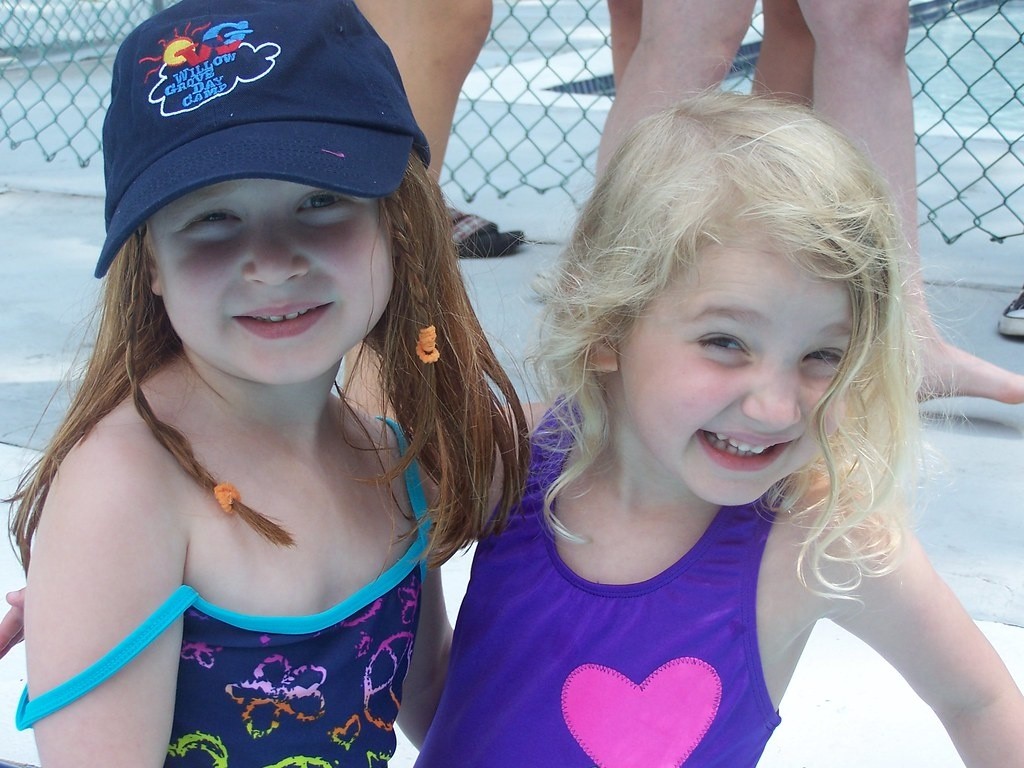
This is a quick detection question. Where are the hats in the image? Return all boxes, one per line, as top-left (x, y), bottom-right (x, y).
top-left (91, 1), bottom-right (429, 279)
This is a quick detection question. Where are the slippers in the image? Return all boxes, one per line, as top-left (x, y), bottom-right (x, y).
top-left (447, 213), bottom-right (527, 260)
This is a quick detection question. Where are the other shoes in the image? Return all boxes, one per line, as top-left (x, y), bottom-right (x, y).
top-left (996, 293), bottom-right (1024, 338)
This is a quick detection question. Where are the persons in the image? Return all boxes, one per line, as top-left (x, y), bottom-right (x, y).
top-left (0, 89), bottom-right (1024, 768)
top-left (593, 0), bottom-right (757, 190)
top-left (751, 1), bottom-right (1023, 404)
top-left (350, 0), bottom-right (528, 261)
top-left (9, 1), bottom-right (540, 768)
